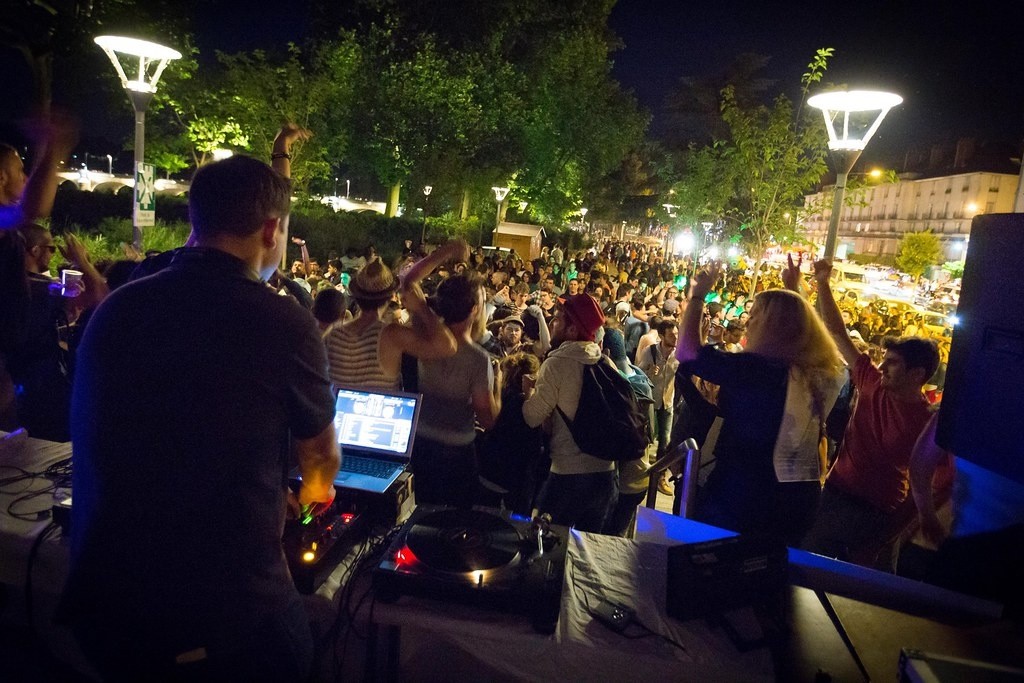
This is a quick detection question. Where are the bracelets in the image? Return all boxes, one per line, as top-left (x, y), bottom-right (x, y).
top-left (692, 296), bottom-right (705, 302)
top-left (271, 151), bottom-right (292, 160)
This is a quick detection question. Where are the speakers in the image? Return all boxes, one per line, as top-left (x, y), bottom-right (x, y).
top-left (934, 212), bottom-right (1024, 487)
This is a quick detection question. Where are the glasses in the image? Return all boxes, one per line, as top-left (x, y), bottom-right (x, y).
top-left (25, 244), bottom-right (57, 254)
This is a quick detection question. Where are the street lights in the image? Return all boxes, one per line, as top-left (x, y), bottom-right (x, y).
top-left (87, 35), bottom-right (183, 257)
top-left (577, 206), bottom-right (589, 227)
top-left (104, 153), bottom-right (113, 177)
top-left (345, 180), bottom-right (351, 200)
top-left (420, 185), bottom-right (434, 248)
top-left (491, 187), bottom-right (514, 249)
top-left (804, 88), bottom-right (904, 312)
top-left (700, 218), bottom-right (712, 261)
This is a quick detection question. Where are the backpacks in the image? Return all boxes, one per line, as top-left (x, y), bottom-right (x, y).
top-left (555, 354), bottom-right (652, 461)
top-left (603, 301), bottom-right (629, 324)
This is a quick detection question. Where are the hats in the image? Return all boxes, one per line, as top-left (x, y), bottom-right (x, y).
top-left (347, 256), bottom-right (401, 301)
top-left (554, 291), bottom-right (606, 342)
top-left (663, 299), bottom-right (680, 311)
top-left (502, 315), bottom-right (526, 329)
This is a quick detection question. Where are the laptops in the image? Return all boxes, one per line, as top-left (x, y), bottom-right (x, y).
top-left (287, 382), bottom-right (424, 493)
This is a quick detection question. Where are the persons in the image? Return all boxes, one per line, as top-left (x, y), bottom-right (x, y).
top-left (266, 121), bottom-right (1024, 606)
top-left (0, 106), bottom-right (135, 441)
top-left (72, 155), bottom-right (341, 683)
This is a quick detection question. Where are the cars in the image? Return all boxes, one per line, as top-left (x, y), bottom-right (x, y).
top-left (828, 262), bottom-right (962, 349)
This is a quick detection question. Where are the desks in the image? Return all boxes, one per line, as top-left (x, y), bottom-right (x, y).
top-left (0, 429), bottom-right (1024, 683)
